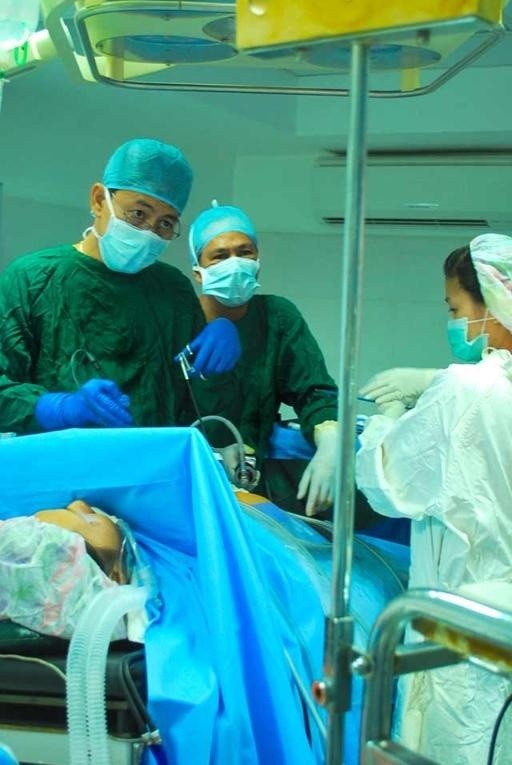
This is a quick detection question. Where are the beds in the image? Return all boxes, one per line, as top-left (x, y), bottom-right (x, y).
top-left (2, 621), bottom-right (146, 765)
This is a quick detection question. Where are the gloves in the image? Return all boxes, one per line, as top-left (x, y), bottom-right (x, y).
top-left (296, 420), bottom-right (338, 517)
top-left (359, 367), bottom-right (437, 407)
top-left (35, 378), bottom-right (135, 431)
top-left (173, 316), bottom-right (242, 379)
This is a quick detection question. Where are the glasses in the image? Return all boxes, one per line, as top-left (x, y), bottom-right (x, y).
top-left (112, 192), bottom-right (181, 241)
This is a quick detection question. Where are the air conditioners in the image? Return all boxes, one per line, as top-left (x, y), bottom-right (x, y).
top-left (314, 154), bottom-right (510, 238)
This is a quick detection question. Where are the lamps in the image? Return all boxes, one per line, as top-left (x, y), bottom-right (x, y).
top-left (40, 0), bottom-right (504, 98)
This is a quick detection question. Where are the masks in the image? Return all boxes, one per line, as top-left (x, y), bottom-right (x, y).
top-left (199, 260), bottom-right (262, 308)
top-left (447, 320), bottom-right (489, 364)
top-left (95, 218), bottom-right (172, 274)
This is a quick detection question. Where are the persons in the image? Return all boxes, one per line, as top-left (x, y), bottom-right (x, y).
top-left (1, 139), bottom-right (246, 434)
top-left (355, 231), bottom-right (512, 765)
top-left (0, 498), bottom-right (144, 647)
top-left (187, 207), bottom-right (338, 518)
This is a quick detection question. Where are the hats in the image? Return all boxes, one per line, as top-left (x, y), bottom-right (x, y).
top-left (103, 138), bottom-right (194, 211)
top-left (188, 205), bottom-right (259, 268)
